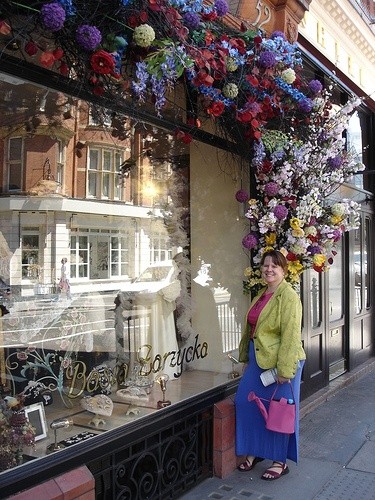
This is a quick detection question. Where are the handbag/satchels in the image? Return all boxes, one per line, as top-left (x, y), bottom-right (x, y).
top-left (249, 381), bottom-right (295, 434)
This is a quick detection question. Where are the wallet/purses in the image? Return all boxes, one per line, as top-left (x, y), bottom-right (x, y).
top-left (260, 368), bottom-right (277, 387)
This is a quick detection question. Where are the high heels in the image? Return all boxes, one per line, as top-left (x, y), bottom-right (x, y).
top-left (238, 456), bottom-right (258, 472)
top-left (262, 461), bottom-right (289, 480)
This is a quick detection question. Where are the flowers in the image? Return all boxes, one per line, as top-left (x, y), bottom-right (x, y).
top-left (2, 2), bottom-right (365, 295)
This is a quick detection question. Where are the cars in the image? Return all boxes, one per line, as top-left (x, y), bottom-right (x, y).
top-left (116, 260), bottom-right (177, 304)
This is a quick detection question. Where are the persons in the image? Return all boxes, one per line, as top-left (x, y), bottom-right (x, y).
top-left (234, 250), bottom-right (307, 480)
top-left (52, 258), bottom-right (73, 300)
